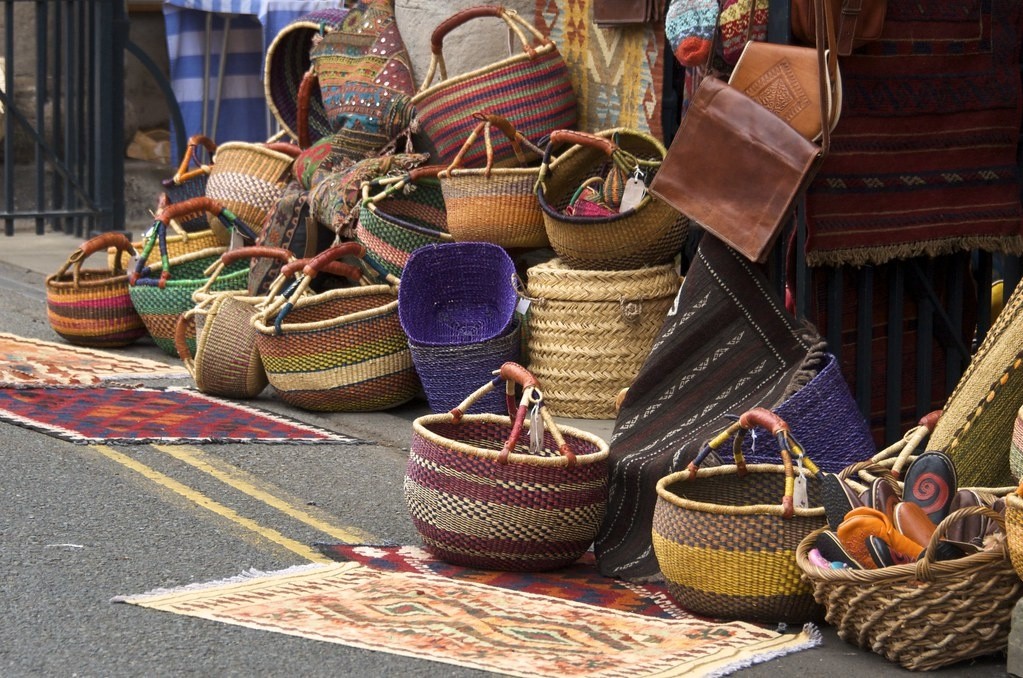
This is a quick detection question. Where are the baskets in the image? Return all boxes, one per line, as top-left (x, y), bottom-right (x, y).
top-left (1009, 410), bottom-right (1022, 478)
top-left (719, 352), bottom-right (880, 480)
top-left (794, 492), bottom-right (1014, 672)
top-left (1002, 487), bottom-right (1022, 580)
top-left (400, 361), bottom-right (609, 568)
top-left (857, 457), bottom-right (920, 488)
top-left (44, 0), bottom-right (686, 418)
top-left (655, 406), bottom-right (832, 623)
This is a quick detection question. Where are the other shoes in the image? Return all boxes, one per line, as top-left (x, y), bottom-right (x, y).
top-left (814, 452), bottom-right (1008, 563)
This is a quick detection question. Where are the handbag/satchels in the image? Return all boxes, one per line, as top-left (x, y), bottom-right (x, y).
top-left (653, 75), bottom-right (824, 264)
top-left (791, 1), bottom-right (887, 52)
top-left (728, 37), bottom-right (842, 145)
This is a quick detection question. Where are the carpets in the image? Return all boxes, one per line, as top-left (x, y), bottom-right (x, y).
top-left (791, 0), bottom-right (1023, 268)
top-left (104, 559), bottom-right (824, 678)
top-left (0, 333), bottom-right (193, 391)
top-left (0, 386), bottom-right (382, 447)
top-left (593, 228), bottom-right (838, 581)
top-left (310, 540), bottom-right (789, 634)
